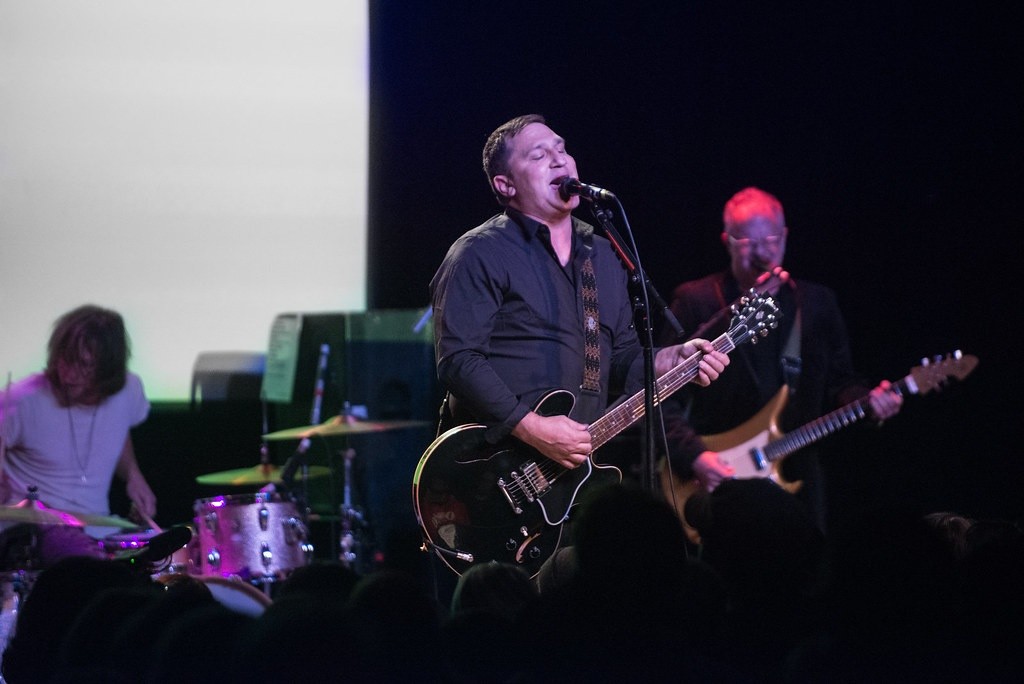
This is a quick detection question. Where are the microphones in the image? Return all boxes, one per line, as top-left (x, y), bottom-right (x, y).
top-left (560, 176), bottom-right (616, 203)
top-left (127, 525), bottom-right (194, 566)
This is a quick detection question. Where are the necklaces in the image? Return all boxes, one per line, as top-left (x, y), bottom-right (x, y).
top-left (64, 392), bottom-right (101, 485)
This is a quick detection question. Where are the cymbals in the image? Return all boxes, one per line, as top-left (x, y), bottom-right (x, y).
top-left (196, 464), bottom-right (336, 487)
top-left (0, 499), bottom-right (138, 530)
top-left (263, 416), bottom-right (425, 439)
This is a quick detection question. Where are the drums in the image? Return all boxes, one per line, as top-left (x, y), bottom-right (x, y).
top-left (194, 494), bottom-right (314, 583)
top-left (151, 567), bottom-right (272, 620)
top-left (104, 532), bottom-right (190, 573)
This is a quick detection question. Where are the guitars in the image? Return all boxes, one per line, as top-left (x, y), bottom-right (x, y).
top-left (664, 350), bottom-right (979, 541)
top-left (412, 290), bottom-right (780, 583)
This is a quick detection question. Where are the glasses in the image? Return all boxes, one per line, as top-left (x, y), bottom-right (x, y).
top-left (729, 235), bottom-right (784, 250)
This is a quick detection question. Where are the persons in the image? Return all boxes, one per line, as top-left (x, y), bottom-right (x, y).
top-left (654, 186), bottom-right (903, 556)
top-left (0, 303), bottom-right (159, 565)
top-left (430, 112), bottom-right (731, 612)
top-left (0, 444), bottom-right (1024, 683)
